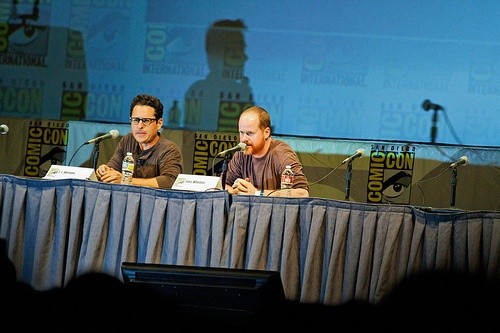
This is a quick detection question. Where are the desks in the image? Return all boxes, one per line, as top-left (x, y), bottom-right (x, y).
top-left (0, 173), bottom-right (500, 307)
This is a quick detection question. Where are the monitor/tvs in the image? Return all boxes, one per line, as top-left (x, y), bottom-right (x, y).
top-left (121, 261), bottom-right (286, 315)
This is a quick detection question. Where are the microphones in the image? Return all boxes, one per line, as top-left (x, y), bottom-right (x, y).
top-left (450, 156), bottom-right (469, 168)
top-left (217, 143), bottom-right (247, 155)
top-left (85, 130), bottom-right (119, 145)
top-left (0, 124), bottom-right (10, 134)
top-left (341, 149), bottom-right (364, 165)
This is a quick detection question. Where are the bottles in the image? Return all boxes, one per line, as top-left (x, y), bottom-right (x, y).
top-left (121, 152), bottom-right (134, 185)
top-left (281, 165), bottom-right (294, 197)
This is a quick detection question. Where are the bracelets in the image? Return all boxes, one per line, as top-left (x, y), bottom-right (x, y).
top-left (260, 189), bottom-right (264, 197)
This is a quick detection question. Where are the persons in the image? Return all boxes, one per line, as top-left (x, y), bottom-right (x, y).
top-left (220, 106), bottom-right (309, 198)
top-left (96, 94), bottom-right (184, 189)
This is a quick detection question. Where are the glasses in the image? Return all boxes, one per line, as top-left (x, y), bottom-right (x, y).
top-left (129, 117), bottom-right (158, 126)
